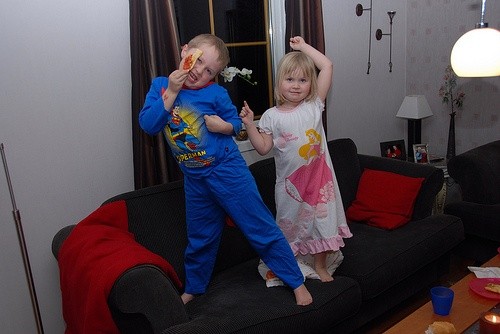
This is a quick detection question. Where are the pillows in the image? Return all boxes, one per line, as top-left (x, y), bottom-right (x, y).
top-left (346, 167), bottom-right (426, 231)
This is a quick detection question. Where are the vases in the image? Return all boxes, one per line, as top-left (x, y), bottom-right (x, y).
top-left (445, 114), bottom-right (457, 164)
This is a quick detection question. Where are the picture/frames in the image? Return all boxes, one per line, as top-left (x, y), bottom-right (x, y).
top-left (380, 139), bottom-right (407, 161)
top-left (413, 144), bottom-right (431, 164)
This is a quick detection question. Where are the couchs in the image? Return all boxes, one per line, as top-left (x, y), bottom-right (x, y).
top-left (443, 140), bottom-right (500, 261)
top-left (52, 138), bottom-right (465, 334)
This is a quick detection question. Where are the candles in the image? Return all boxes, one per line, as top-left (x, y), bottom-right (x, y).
top-left (479, 312), bottom-right (500, 334)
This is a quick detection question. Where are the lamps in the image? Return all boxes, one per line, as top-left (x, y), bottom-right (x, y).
top-left (356, 0), bottom-right (396, 74)
top-left (396, 95), bottom-right (433, 161)
top-left (450, 0), bottom-right (500, 78)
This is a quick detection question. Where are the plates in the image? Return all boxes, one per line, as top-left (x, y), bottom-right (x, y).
top-left (468, 278), bottom-right (500, 299)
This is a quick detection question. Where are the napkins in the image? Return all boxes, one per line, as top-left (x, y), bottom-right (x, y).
top-left (467, 266), bottom-right (500, 278)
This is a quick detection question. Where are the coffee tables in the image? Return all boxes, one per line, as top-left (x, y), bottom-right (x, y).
top-left (383, 254), bottom-right (500, 334)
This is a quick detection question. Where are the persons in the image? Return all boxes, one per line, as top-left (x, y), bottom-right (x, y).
top-left (139, 34), bottom-right (312, 306)
top-left (416, 147), bottom-right (428, 163)
top-left (386, 145), bottom-right (401, 159)
top-left (240, 36), bottom-right (352, 283)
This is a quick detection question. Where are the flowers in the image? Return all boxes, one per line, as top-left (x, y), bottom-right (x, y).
top-left (439, 63), bottom-right (465, 113)
top-left (220, 66), bottom-right (252, 84)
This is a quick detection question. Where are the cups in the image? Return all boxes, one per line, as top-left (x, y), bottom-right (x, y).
top-left (430, 287), bottom-right (454, 316)
